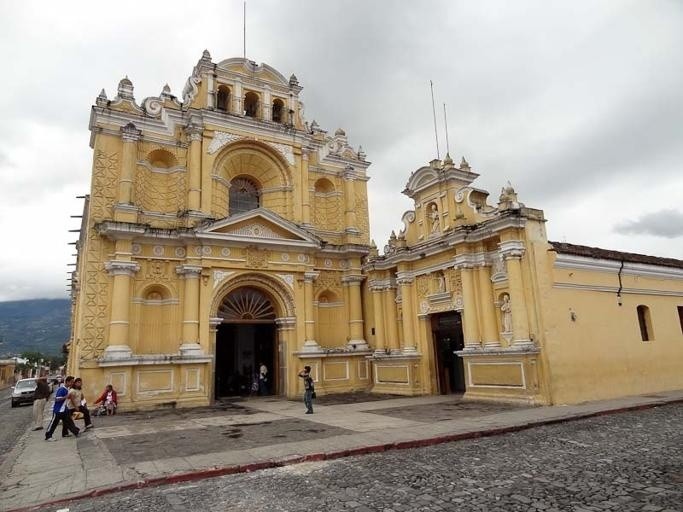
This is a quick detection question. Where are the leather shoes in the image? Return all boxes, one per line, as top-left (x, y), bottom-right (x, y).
top-left (305, 411), bottom-right (313, 414)
top-left (31, 426), bottom-right (43, 431)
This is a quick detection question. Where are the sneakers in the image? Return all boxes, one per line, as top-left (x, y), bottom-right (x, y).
top-left (45, 423), bottom-right (93, 442)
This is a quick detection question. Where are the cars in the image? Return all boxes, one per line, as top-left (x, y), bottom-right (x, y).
top-left (10, 374), bottom-right (60, 407)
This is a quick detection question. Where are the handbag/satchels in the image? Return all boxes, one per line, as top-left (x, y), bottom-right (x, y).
top-left (312, 392), bottom-right (316, 398)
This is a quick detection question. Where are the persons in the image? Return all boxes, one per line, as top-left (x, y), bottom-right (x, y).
top-left (259, 361), bottom-right (268, 395)
top-left (431, 205), bottom-right (439, 233)
top-left (94, 385), bottom-right (116, 416)
top-left (501, 295), bottom-right (512, 333)
top-left (45, 376), bottom-right (94, 441)
top-left (438, 273), bottom-right (444, 288)
top-left (298, 366), bottom-right (314, 414)
